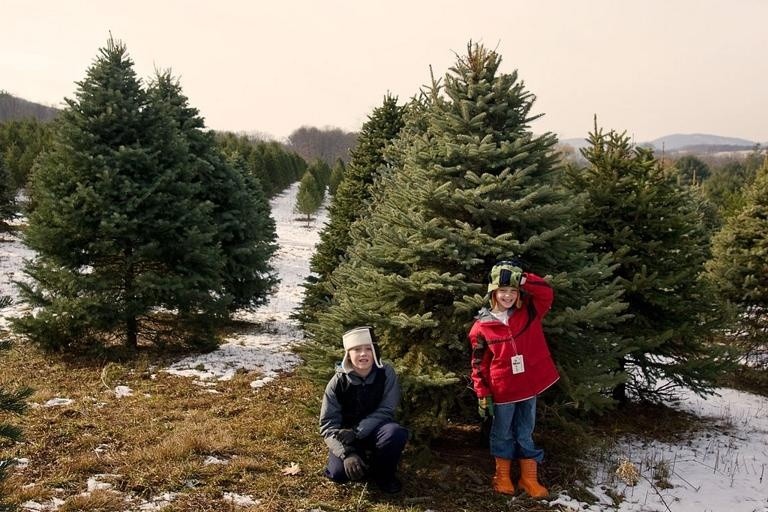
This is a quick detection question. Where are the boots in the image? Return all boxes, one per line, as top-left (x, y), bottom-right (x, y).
top-left (492, 457), bottom-right (515, 493)
top-left (519, 460), bottom-right (549, 499)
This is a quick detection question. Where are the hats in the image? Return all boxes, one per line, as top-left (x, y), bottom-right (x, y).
top-left (342, 326), bottom-right (383, 374)
top-left (488, 261), bottom-right (523, 309)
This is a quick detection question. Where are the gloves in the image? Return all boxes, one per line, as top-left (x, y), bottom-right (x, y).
top-left (338, 428), bottom-right (357, 449)
top-left (478, 397), bottom-right (495, 419)
top-left (343, 454), bottom-right (368, 482)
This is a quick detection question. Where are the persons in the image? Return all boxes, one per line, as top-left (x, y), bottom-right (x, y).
top-left (468, 258), bottom-right (561, 498)
top-left (317, 325), bottom-right (409, 495)
